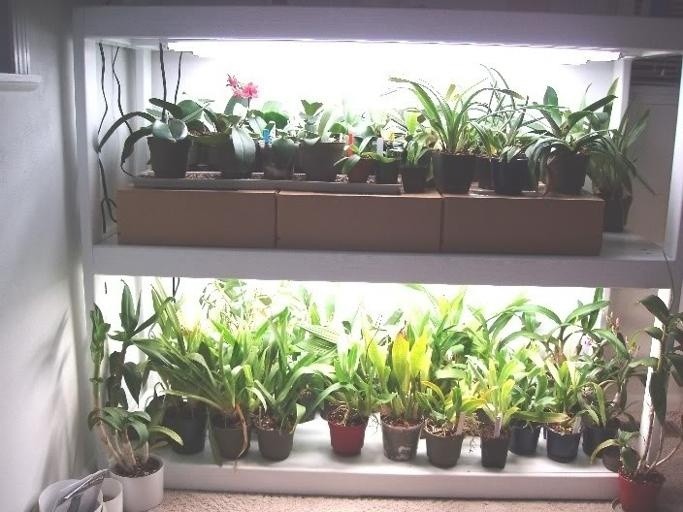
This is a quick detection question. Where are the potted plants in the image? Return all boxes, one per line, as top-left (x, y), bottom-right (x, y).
top-left (414, 372), bottom-right (476, 467)
top-left (333, 134), bottom-right (394, 184)
top-left (401, 115), bottom-right (434, 193)
top-left (379, 76), bottom-right (526, 193)
top-left (260, 128), bottom-right (297, 179)
top-left (278, 322), bottom-right (378, 456)
top-left (80, 276), bottom-right (168, 512)
top-left (370, 143), bottom-right (401, 186)
top-left (300, 99), bottom-right (345, 181)
top-left (479, 85), bottom-right (523, 196)
top-left (247, 300), bottom-right (315, 461)
top-left (193, 103), bottom-right (259, 177)
top-left (143, 276), bottom-right (223, 469)
top-left (526, 84), bottom-right (621, 195)
top-left (160, 316), bottom-right (253, 460)
top-left (150, 97), bottom-right (210, 170)
top-left (462, 308), bottom-right (546, 470)
top-left (585, 315), bottom-right (657, 460)
top-left (490, 104), bottom-right (566, 195)
top-left (507, 293), bottom-right (544, 458)
top-left (617, 293), bottom-right (682, 510)
top-left (579, 77), bottom-right (659, 231)
top-left (93, 105), bottom-right (210, 177)
top-left (364, 308), bottom-right (436, 462)
top-left (472, 63), bottom-right (543, 193)
top-left (543, 325), bottom-right (606, 464)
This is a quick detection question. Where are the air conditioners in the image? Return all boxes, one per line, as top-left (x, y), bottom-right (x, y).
top-left (623, 52), bottom-right (683, 259)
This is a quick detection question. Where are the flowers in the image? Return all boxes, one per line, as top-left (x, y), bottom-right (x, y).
top-left (218, 73), bottom-right (259, 114)
